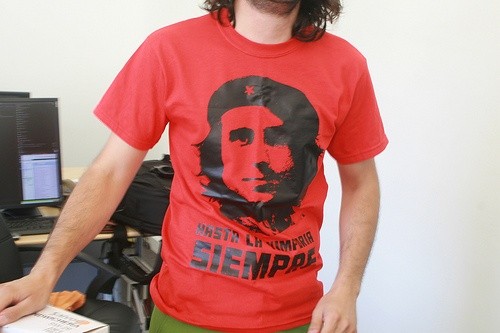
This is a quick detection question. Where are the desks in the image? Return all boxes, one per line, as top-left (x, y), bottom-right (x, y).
top-left (13, 167), bottom-right (160, 246)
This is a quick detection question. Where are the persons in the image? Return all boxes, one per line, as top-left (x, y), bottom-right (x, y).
top-left (0, 0), bottom-right (390, 333)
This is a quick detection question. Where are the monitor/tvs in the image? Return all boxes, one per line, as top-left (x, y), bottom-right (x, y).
top-left (0, 98), bottom-right (64, 219)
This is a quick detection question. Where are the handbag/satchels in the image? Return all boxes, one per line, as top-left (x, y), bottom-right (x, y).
top-left (111, 154), bottom-right (177, 285)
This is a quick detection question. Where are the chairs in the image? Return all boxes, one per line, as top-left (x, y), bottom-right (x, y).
top-left (0, 214), bottom-right (163, 333)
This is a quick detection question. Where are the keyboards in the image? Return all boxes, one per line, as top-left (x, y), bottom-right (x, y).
top-left (6, 216), bottom-right (59, 236)
